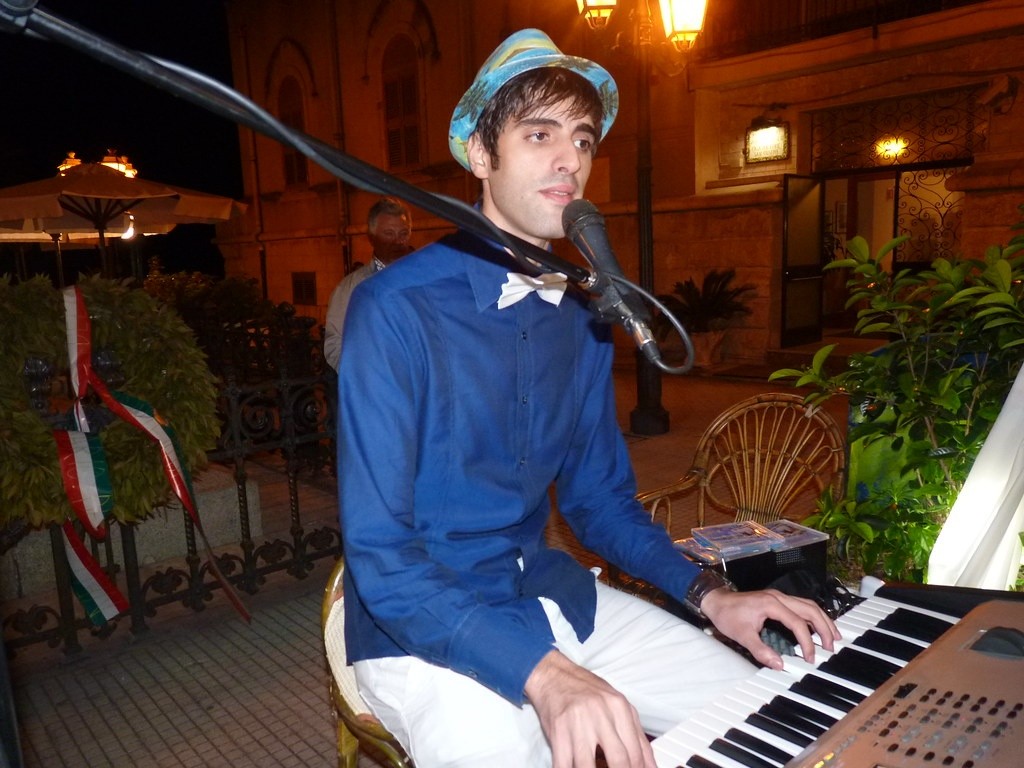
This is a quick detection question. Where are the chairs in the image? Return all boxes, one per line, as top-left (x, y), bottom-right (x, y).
top-left (606, 392), bottom-right (846, 605)
top-left (320, 558), bottom-right (416, 768)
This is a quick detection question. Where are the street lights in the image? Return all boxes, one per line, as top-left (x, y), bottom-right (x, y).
top-left (574, 0), bottom-right (711, 434)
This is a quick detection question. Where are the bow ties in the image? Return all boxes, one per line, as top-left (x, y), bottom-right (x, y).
top-left (497, 273), bottom-right (566, 310)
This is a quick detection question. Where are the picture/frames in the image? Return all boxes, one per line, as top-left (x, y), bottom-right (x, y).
top-left (835, 200), bottom-right (847, 234)
top-left (825, 210), bottom-right (833, 231)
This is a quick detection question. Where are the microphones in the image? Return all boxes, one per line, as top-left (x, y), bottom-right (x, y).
top-left (561, 199), bottom-right (663, 363)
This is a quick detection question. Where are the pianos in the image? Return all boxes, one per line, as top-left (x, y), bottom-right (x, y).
top-left (650, 583), bottom-right (1024, 768)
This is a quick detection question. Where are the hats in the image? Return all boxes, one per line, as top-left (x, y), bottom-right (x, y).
top-left (448, 27), bottom-right (619, 173)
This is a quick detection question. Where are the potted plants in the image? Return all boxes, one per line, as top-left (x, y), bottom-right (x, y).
top-left (656, 268), bottom-right (758, 367)
top-left (823, 214), bottom-right (844, 316)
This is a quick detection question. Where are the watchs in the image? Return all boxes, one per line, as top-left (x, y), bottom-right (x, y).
top-left (685, 569), bottom-right (737, 619)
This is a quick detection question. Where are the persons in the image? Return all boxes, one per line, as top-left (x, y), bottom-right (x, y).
top-left (324, 197), bottom-right (412, 374)
top-left (336, 26), bottom-right (840, 767)
